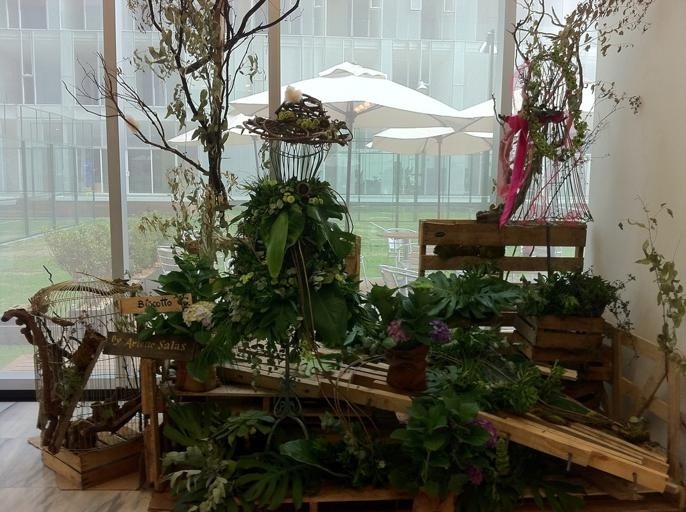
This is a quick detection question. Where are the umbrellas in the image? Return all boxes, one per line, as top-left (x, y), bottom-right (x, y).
top-left (166, 106), bottom-right (269, 183)
top-left (456, 80), bottom-right (595, 134)
top-left (225, 58), bottom-right (471, 231)
top-left (364, 125), bottom-right (493, 221)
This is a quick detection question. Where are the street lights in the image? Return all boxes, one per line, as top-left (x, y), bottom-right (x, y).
top-left (480, 29), bottom-right (497, 202)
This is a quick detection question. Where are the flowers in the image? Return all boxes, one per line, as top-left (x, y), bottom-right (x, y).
top-left (364, 281), bottom-right (452, 349)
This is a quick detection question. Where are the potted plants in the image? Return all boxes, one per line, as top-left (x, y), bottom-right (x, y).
top-left (513, 264), bottom-right (640, 366)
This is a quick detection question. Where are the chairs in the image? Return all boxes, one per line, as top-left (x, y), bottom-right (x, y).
top-left (157, 230), bottom-right (234, 277)
top-left (381, 227), bottom-right (419, 296)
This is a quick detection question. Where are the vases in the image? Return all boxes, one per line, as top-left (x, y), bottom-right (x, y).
top-left (387, 344), bottom-right (429, 391)
